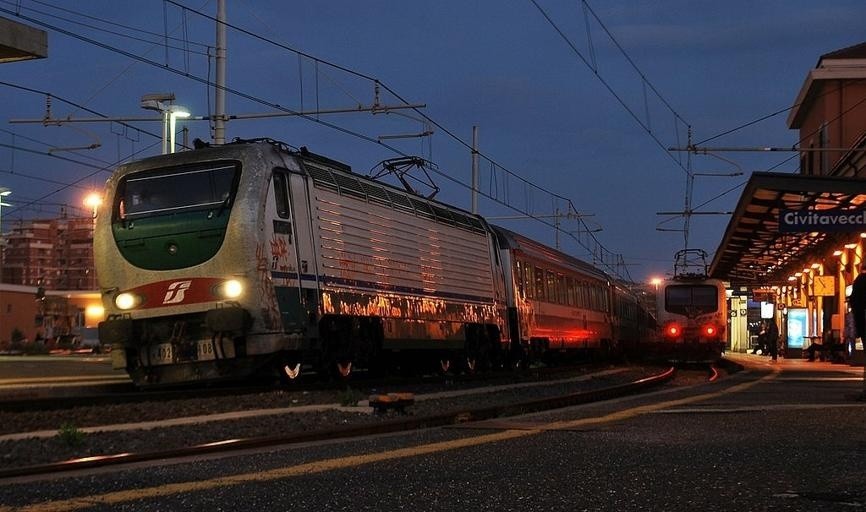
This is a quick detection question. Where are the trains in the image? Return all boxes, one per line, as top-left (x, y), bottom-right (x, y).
top-left (655, 274), bottom-right (729, 366)
top-left (91, 136), bottom-right (645, 376)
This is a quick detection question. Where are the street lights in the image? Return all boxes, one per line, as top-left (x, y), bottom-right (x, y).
top-left (137, 100), bottom-right (192, 152)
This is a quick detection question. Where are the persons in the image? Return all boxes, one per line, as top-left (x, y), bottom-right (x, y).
top-left (751, 318), bottom-right (833, 362)
